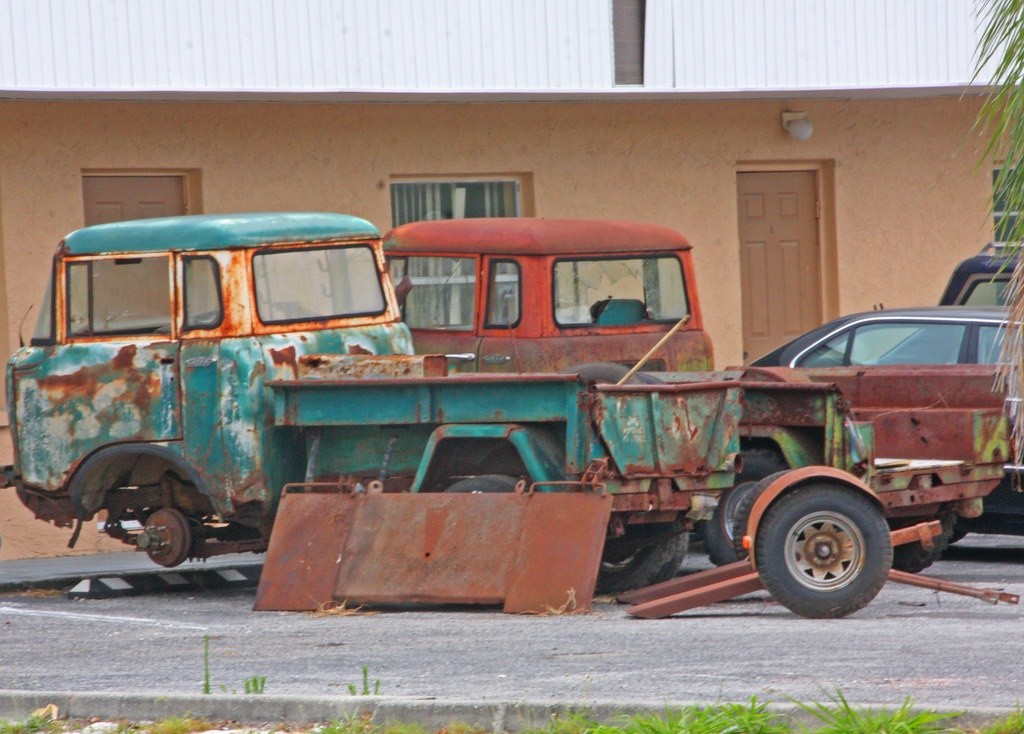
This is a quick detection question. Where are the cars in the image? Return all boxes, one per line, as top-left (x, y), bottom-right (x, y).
top-left (739, 303), bottom-right (1024, 544)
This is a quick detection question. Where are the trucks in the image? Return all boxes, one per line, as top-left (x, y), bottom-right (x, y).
top-left (0, 213), bottom-right (1013, 622)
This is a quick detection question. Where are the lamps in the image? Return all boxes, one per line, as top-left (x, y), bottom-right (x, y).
top-left (783, 111), bottom-right (813, 141)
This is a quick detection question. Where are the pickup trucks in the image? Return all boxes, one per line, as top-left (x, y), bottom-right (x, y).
top-left (877, 239), bottom-right (1024, 367)
